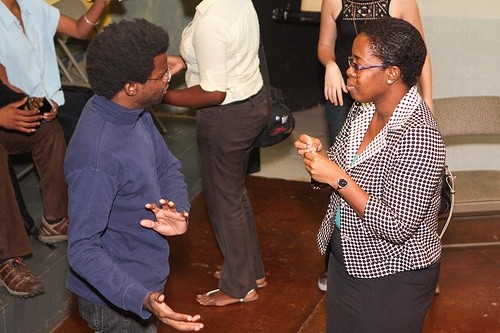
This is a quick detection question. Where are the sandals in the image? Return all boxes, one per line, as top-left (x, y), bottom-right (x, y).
top-left (213, 270), bottom-right (267, 288)
top-left (208, 286), bottom-right (259, 305)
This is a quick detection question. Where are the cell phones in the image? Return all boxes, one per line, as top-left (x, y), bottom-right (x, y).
top-left (38, 95), bottom-right (55, 123)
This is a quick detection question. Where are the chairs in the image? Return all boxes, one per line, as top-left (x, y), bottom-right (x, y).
top-left (53, 0), bottom-right (164, 132)
top-left (432, 95), bottom-right (500, 293)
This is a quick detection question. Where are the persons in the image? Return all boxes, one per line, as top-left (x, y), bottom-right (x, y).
top-left (317, 0), bottom-right (435, 146)
top-left (0, 0), bottom-right (108, 123)
top-left (0, 78), bottom-right (69, 300)
top-left (62, 19), bottom-right (204, 333)
top-left (160, 0), bottom-right (271, 306)
top-left (294, 17), bottom-right (446, 333)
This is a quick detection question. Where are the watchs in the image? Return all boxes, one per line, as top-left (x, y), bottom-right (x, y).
top-left (336, 178), bottom-right (348, 189)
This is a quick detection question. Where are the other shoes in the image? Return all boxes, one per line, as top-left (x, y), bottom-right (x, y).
top-left (318, 269), bottom-right (328, 292)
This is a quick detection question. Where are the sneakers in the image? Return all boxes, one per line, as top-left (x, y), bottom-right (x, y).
top-left (0, 257), bottom-right (44, 298)
top-left (37, 216), bottom-right (69, 243)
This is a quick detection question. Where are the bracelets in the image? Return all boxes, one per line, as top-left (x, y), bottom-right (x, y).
top-left (84, 13), bottom-right (100, 25)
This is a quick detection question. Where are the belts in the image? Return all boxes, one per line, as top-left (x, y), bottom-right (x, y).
top-left (230, 88), bottom-right (263, 105)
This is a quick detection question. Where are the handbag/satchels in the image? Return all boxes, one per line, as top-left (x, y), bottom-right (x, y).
top-left (256, 102), bottom-right (295, 146)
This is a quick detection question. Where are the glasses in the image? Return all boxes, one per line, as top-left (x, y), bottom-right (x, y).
top-left (347, 56), bottom-right (388, 73)
